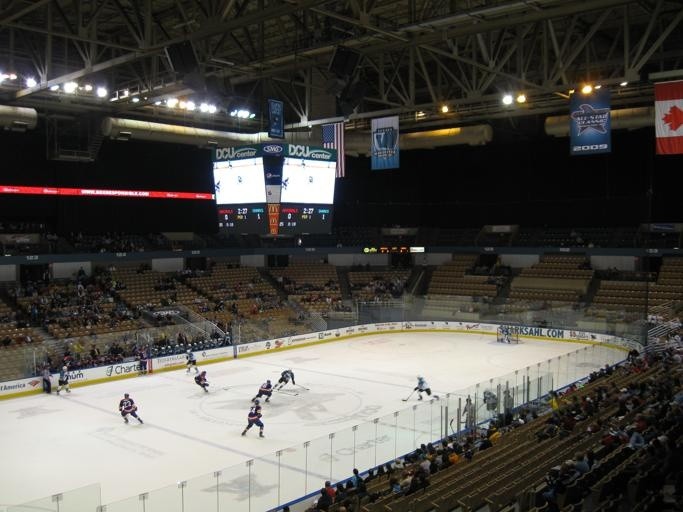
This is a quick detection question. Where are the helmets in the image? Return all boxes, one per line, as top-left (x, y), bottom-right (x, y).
top-left (62, 366), bottom-right (67, 370)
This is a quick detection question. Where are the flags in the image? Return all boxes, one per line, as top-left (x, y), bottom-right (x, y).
top-left (371, 116), bottom-right (400, 170)
top-left (570, 89), bottom-right (612, 154)
top-left (654, 79), bottom-right (683, 154)
top-left (322, 124), bottom-right (345, 178)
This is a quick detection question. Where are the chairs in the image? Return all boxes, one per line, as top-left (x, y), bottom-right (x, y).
top-left (304, 423), bottom-right (515, 510)
top-left (515, 358), bottom-right (683, 510)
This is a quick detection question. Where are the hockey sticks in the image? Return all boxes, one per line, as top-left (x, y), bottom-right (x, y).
top-left (274, 390), bottom-right (298, 397)
top-left (294, 383), bottom-right (310, 390)
top-left (450, 418), bottom-right (456, 436)
top-left (402, 390), bottom-right (415, 401)
